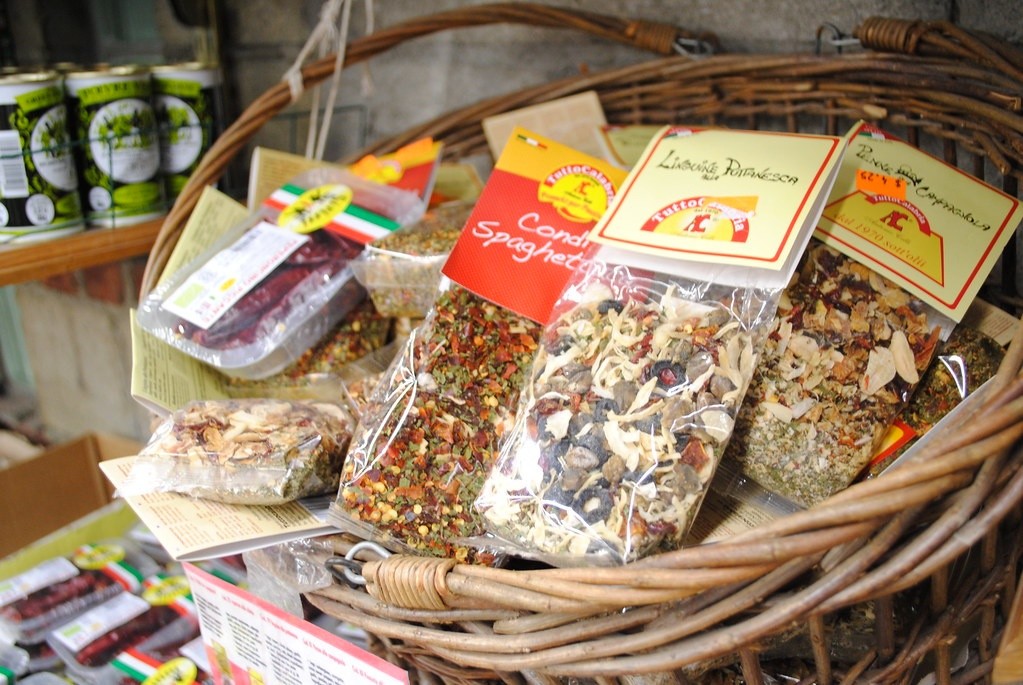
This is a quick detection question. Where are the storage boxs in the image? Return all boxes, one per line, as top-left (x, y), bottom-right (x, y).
top-left (0, 430), bottom-right (145, 563)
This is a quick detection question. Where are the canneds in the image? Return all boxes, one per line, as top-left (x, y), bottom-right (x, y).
top-left (0, 65), bottom-right (219, 244)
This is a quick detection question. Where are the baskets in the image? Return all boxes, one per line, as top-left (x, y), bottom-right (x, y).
top-left (134, 0), bottom-right (1019, 682)
